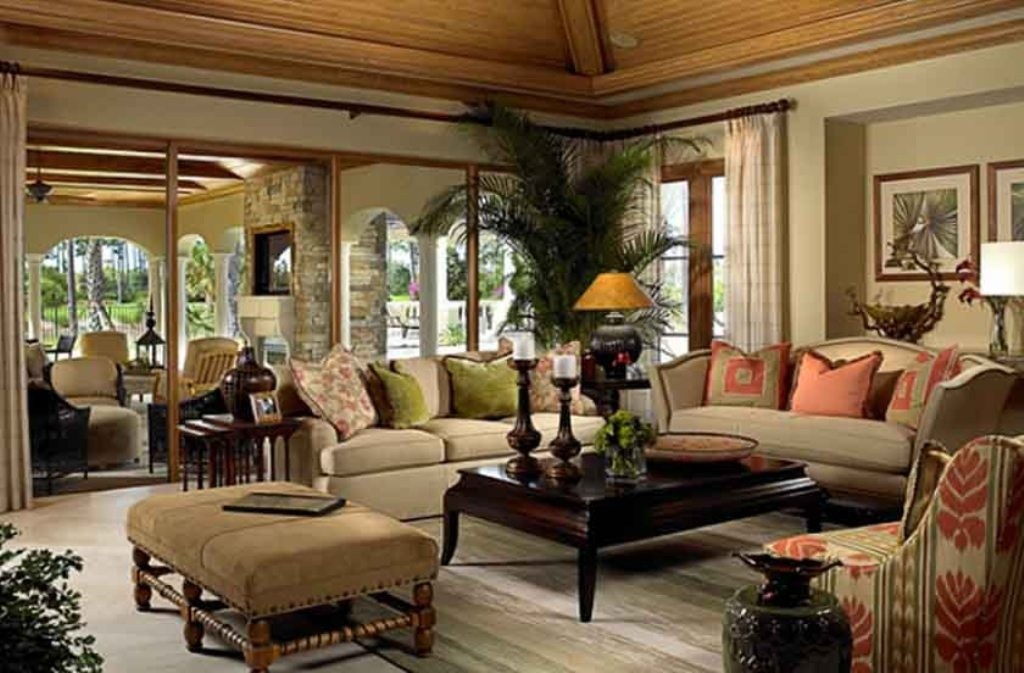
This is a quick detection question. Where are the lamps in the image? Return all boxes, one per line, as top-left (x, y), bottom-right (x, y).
top-left (26, 153), bottom-right (52, 203)
top-left (571, 266), bottom-right (655, 381)
top-left (979, 242), bottom-right (1024, 363)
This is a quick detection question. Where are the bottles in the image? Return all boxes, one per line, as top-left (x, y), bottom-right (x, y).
top-left (222, 345), bottom-right (276, 423)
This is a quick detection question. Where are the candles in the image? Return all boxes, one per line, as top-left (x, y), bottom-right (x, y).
top-left (552, 353), bottom-right (576, 378)
top-left (511, 331), bottom-right (536, 360)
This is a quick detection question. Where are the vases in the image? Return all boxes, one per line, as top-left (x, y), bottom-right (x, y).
top-left (722, 551), bottom-right (852, 673)
top-left (220, 346), bottom-right (277, 419)
top-left (984, 309), bottom-right (1015, 360)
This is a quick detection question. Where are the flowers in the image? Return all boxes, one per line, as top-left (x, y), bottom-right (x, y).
top-left (956, 252), bottom-right (1023, 352)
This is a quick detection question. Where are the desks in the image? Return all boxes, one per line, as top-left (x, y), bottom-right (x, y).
top-left (120, 369), bottom-right (157, 408)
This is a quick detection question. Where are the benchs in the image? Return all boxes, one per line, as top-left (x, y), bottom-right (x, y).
top-left (127, 476), bottom-right (438, 673)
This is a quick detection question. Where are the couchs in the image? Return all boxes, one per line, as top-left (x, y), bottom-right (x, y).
top-left (258, 349), bottom-right (608, 520)
top-left (646, 334), bottom-right (1018, 504)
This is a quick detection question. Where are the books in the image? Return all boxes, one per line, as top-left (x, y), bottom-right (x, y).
top-left (222, 492), bottom-right (346, 516)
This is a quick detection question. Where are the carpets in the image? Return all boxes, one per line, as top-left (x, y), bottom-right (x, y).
top-left (342, 513), bottom-right (849, 673)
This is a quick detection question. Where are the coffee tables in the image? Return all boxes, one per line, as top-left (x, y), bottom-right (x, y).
top-left (440, 450), bottom-right (825, 623)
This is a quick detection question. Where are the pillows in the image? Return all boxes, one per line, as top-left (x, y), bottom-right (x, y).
top-left (287, 342), bottom-right (380, 441)
top-left (897, 438), bottom-right (952, 542)
top-left (442, 352), bottom-right (518, 420)
top-left (703, 339), bottom-right (792, 413)
top-left (497, 337), bottom-right (585, 416)
top-left (367, 362), bottom-right (429, 429)
top-left (883, 345), bottom-right (962, 432)
top-left (785, 347), bottom-right (883, 420)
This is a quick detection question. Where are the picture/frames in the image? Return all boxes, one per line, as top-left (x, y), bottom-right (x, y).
top-left (248, 391), bottom-right (282, 427)
top-left (221, 492), bottom-right (346, 516)
top-left (988, 157), bottom-right (1024, 242)
top-left (872, 163), bottom-right (980, 282)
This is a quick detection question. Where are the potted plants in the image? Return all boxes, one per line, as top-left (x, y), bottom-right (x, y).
top-left (405, 86), bottom-right (715, 418)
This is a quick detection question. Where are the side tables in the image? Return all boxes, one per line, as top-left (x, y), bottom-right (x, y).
top-left (580, 380), bottom-right (651, 415)
top-left (176, 412), bottom-right (301, 491)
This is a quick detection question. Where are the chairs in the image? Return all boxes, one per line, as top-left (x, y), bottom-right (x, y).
top-left (762, 436), bottom-right (1024, 673)
top-left (26, 333), bottom-right (237, 497)
